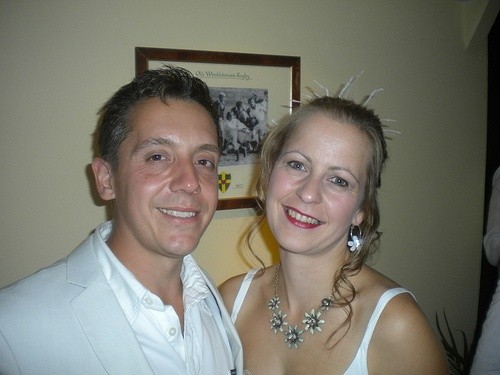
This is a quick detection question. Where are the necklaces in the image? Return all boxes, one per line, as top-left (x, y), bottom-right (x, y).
top-left (268, 257), bottom-right (345, 348)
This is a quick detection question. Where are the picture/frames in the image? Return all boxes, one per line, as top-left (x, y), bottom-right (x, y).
top-left (134, 46), bottom-right (303, 213)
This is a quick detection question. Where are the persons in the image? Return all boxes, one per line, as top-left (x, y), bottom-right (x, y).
top-left (219, 92), bottom-right (454, 375)
top-left (0, 62), bottom-right (241, 374)
top-left (208, 86), bottom-right (268, 164)
